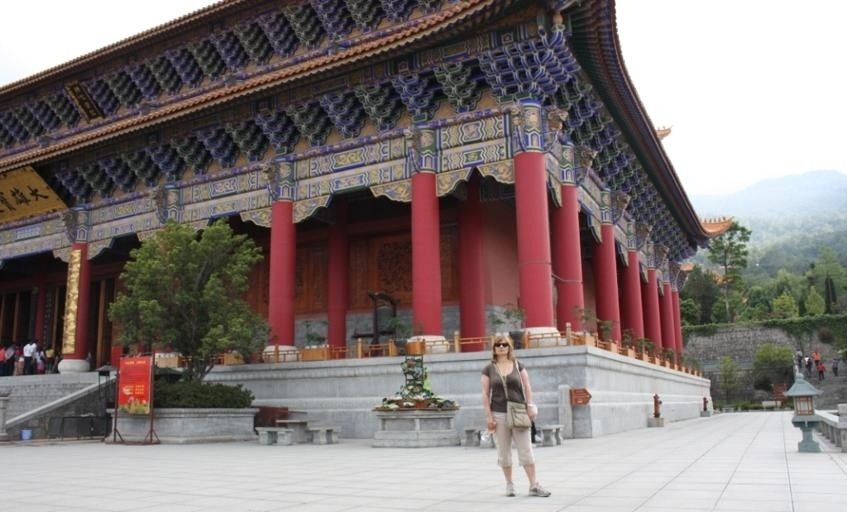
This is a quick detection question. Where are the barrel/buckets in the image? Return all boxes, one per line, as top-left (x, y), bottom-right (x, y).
top-left (20, 429), bottom-right (32, 440)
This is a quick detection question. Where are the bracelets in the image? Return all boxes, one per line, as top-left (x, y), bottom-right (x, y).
top-left (527, 402), bottom-right (536, 407)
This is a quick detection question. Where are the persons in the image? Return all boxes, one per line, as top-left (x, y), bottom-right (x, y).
top-left (831, 357), bottom-right (839, 376)
top-left (803, 355), bottom-right (808, 371)
top-left (816, 361), bottom-right (826, 380)
top-left (478, 331), bottom-right (554, 498)
top-left (811, 350), bottom-right (821, 366)
top-left (0, 337), bottom-right (58, 376)
top-left (807, 358), bottom-right (812, 375)
top-left (795, 347), bottom-right (803, 369)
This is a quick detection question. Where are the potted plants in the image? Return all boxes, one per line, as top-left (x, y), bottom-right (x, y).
top-left (300, 317), bottom-right (333, 361)
top-left (571, 302), bottom-right (704, 378)
top-left (486, 301), bottom-right (528, 349)
top-left (386, 315), bottom-right (413, 356)
top-left (104, 213), bottom-right (275, 444)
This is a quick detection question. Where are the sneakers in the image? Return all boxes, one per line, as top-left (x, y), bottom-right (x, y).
top-left (505, 482), bottom-right (516, 497)
top-left (528, 484), bottom-right (551, 497)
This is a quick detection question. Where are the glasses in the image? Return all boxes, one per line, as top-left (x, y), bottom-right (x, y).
top-left (495, 343), bottom-right (509, 348)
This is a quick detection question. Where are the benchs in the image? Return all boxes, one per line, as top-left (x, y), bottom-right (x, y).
top-left (464, 426), bottom-right (495, 447)
top-left (254, 426), bottom-right (295, 445)
top-left (304, 425), bottom-right (340, 444)
top-left (537, 424), bottom-right (565, 445)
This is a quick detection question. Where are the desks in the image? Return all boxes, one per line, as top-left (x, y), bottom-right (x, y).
top-left (273, 418), bottom-right (311, 444)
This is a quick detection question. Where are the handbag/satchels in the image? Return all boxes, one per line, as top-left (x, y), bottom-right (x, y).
top-left (505, 400), bottom-right (533, 431)
